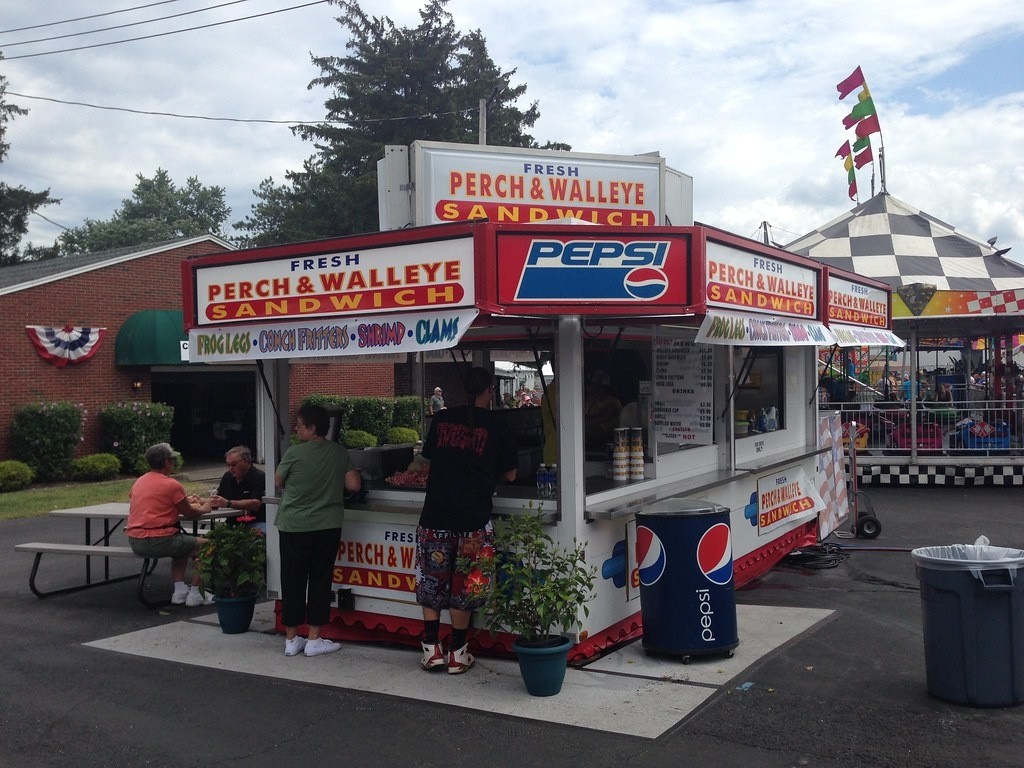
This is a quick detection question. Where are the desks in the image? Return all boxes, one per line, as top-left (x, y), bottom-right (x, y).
top-left (50, 503), bottom-right (245, 587)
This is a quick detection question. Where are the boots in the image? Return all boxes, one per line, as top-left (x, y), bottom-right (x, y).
top-left (420, 640), bottom-right (445, 668)
top-left (448, 643), bottom-right (475, 673)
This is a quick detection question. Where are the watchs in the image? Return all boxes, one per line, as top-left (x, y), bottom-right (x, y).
top-left (226, 500), bottom-right (232, 508)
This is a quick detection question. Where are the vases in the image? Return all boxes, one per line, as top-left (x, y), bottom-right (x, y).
top-left (212, 594), bottom-right (255, 634)
top-left (514, 634), bottom-right (573, 697)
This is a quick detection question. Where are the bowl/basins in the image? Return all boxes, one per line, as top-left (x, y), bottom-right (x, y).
top-left (735, 409), bottom-right (751, 434)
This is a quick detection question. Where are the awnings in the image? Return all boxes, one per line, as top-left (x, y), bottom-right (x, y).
top-left (114, 309), bottom-right (191, 366)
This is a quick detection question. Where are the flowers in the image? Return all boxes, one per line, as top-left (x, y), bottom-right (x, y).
top-left (192, 515), bottom-right (267, 599)
top-left (455, 501), bottom-right (600, 648)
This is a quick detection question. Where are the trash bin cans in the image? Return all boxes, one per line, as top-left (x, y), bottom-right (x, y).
top-left (635, 497), bottom-right (739, 664)
top-left (911, 545), bottom-right (1024, 708)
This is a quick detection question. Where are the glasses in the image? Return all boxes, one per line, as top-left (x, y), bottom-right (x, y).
top-left (487, 385), bottom-right (495, 389)
top-left (225, 458), bottom-right (246, 467)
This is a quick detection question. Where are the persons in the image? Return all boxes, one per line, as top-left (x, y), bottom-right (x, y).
top-left (192, 446), bottom-right (265, 538)
top-left (971, 370), bottom-right (1024, 399)
top-left (415, 368), bottom-right (519, 673)
top-left (125, 443), bottom-right (215, 606)
top-left (502, 385), bottom-right (541, 408)
top-left (428, 387), bottom-right (444, 415)
top-left (542, 355), bottom-right (623, 472)
top-left (877, 373), bottom-right (953, 408)
top-left (274, 405), bottom-right (361, 657)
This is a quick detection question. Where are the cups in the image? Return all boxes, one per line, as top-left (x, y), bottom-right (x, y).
top-left (605, 427), bottom-right (645, 480)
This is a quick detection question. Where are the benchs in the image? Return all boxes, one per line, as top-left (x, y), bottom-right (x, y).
top-left (14, 543), bottom-right (157, 605)
top-left (124, 525), bottom-right (213, 538)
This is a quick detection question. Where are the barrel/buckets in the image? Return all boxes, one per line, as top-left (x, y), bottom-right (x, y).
top-left (637, 498), bottom-right (740, 662)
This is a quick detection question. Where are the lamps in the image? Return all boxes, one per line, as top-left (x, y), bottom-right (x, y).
top-left (131, 374), bottom-right (143, 391)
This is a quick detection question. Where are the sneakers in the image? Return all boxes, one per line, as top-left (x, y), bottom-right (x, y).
top-left (186, 588), bottom-right (215, 606)
top-left (285, 636), bottom-right (308, 655)
top-left (304, 638), bottom-right (341, 657)
top-left (172, 585), bottom-right (190, 604)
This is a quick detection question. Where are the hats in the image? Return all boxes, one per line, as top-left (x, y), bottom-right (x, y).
top-left (434, 387), bottom-right (442, 392)
top-left (503, 393), bottom-right (510, 396)
top-left (525, 396), bottom-right (530, 401)
top-left (146, 442), bottom-right (182, 461)
top-left (521, 392), bottom-right (527, 397)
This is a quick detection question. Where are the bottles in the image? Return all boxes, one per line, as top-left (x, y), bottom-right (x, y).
top-left (536, 463), bottom-right (549, 500)
top-left (548, 464), bottom-right (557, 501)
top-left (210, 486), bottom-right (218, 511)
top-left (749, 405), bottom-right (784, 433)
